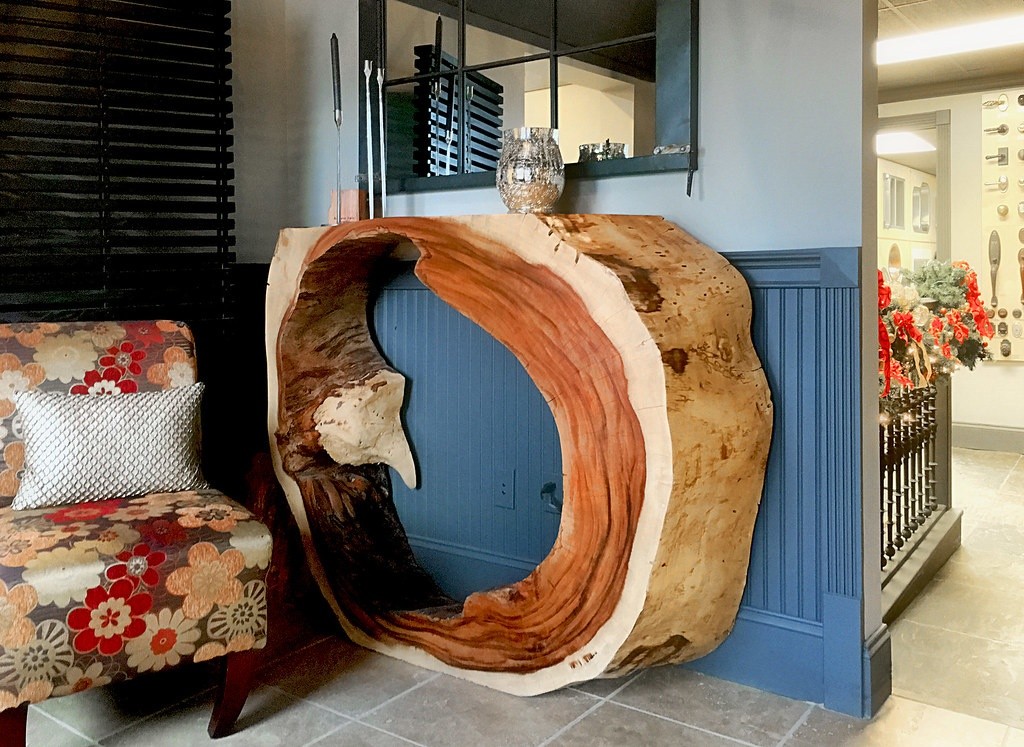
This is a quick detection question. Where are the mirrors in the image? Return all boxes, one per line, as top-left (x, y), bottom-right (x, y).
top-left (359, 0), bottom-right (698, 194)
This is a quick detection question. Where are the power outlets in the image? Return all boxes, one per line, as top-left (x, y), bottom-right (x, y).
top-left (541, 472), bottom-right (563, 513)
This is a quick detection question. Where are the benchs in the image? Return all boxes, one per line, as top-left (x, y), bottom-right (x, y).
top-left (0, 319), bottom-right (274, 747)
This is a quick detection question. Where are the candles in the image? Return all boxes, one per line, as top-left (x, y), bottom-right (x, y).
top-left (330, 33), bottom-right (341, 110)
top-left (447, 65), bottom-right (455, 130)
top-left (434, 12), bottom-right (443, 81)
top-left (377, 0), bottom-right (385, 68)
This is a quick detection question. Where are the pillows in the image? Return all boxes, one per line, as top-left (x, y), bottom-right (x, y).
top-left (9, 383), bottom-right (212, 513)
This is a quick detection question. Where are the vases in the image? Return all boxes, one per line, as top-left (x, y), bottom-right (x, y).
top-left (497, 128), bottom-right (564, 214)
top-left (578, 142), bottom-right (626, 162)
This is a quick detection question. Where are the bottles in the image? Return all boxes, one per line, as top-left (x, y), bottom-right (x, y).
top-left (495, 126), bottom-right (565, 216)
top-left (577, 142), bottom-right (626, 162)
